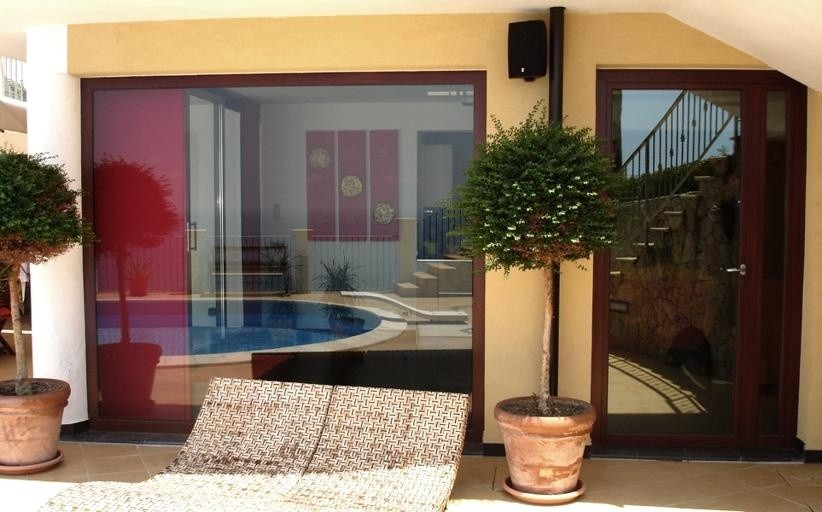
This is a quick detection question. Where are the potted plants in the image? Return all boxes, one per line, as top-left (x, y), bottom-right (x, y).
top-left (311, 255), bottom-right (366, 337)
top-left (95, 153), bottom-right (183, 414)
top-left (253, 239), bottom-right (305, 328)
top-left (0, 143), bottom-right (102, 475)
top-left (438, 98), bottom-right (634, 506)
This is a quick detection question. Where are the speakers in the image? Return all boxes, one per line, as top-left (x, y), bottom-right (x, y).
top-left (506, 19), bottom-right (548, 79)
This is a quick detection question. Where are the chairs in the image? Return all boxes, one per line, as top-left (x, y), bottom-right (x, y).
top-left (222, 385), bottom-right (472, 512)
top-left (36, 377), bottom-right (333, 512)
